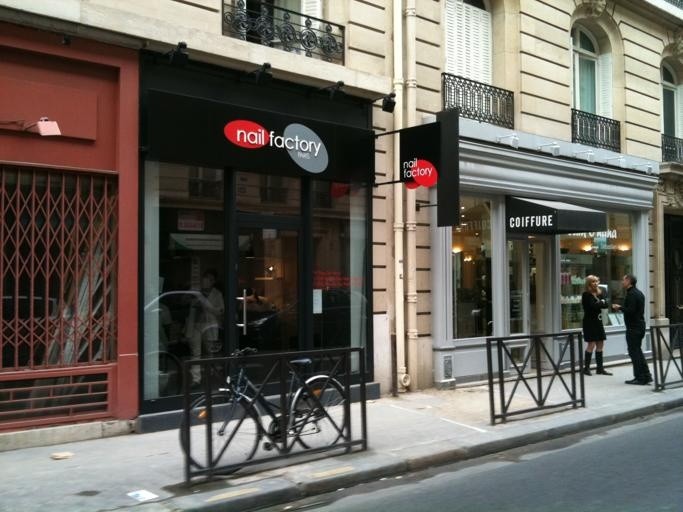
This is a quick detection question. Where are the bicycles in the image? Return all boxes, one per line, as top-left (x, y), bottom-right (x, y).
top-left (179, 347), bottom-right (349, 473)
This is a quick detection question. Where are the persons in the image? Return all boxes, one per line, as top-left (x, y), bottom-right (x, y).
top-left (180, 270), bottom-right (224, 389)
top-left (581, 274), bottom-right (613, 376)
top-left (610, 274), bottom-right (652, 385)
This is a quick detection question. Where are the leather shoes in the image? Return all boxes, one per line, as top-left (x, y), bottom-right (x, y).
top-left (624, 376), bottom-right (654, 385)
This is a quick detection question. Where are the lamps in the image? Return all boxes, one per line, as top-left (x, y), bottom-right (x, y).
top-left (159, 41), bottom-right (397, 114)
top-left (496, 133), bottom-right (654, 176)
top-left (21, 115), bottom-right (63, 137)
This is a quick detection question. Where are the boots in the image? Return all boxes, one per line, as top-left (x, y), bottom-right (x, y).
top-left (582, 350), bottom-right (592, 376)
top-left (595, 350), bottom-right (612, 375)
